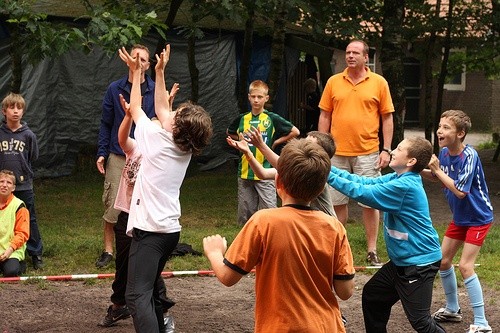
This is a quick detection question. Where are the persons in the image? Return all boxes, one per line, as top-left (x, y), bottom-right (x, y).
top-left (97, 83), bottom-right (183, 327)
top-left (227, 121), bottom-right (348, 329)
top-left (95, 44), bottom-right (164, 254)
top-left (0, 169), bottom-right (30, 280)
top-left (0, 92), bottom-right (44, 270)
top-left (117, 42), bottom-right (212, 332)
top-left (227, 79), bottom-right (301, 229)
top-left (318, 41), bottom-right (396, 267)
top-left (400, 108), bottom-right (494, 333)
top-left (294, 135), bottom-right (450, 333)
top-left (203, 140), bottom-right (355, 333)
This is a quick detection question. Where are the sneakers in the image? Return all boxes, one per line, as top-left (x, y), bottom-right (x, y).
top-left (430, 307), bottom-right (463, 322)
top-left (98, 305), bottom-right (131, 326)
top-left (158, 309), bottom-right (175, 333)
top-left (468, 323), bottom-right (492, 333)
top-left (31, 254), bottom-right (43, 270)
top-left (95, 249), bottom-right (113, 268)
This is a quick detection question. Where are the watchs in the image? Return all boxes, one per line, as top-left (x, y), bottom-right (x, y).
top-left (382, 148), bottom-right (392, 154)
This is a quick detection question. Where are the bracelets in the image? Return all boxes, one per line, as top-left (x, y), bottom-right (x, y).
top-left (246, 154), bottom-right (254, 161)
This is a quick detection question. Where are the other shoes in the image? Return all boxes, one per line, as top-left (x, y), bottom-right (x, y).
top-left (20, 260), bottom-right (26, 275)
top-left (366, 252), bottom-right (380, 266)
